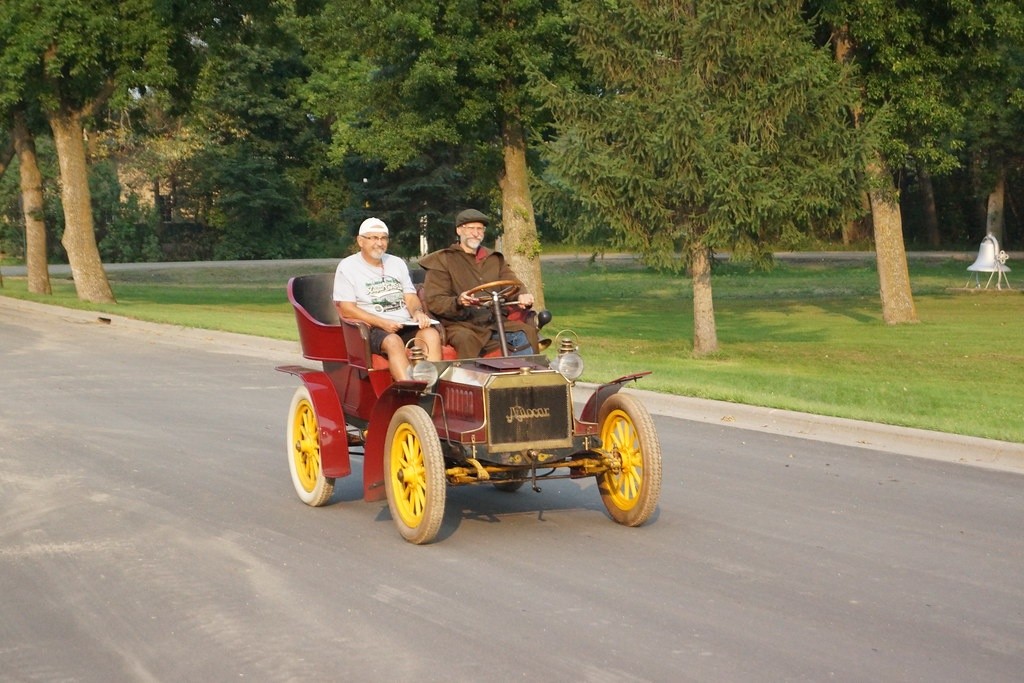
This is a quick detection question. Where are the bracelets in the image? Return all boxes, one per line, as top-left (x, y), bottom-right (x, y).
top-left (412, 307), bottom-right (424, 314)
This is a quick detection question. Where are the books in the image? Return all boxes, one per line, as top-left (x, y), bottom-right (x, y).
top-left (400, 319), bottom-right (440, 327)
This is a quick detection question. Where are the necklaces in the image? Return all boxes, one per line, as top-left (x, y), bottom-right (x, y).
top-left (361, 256), bottom-right (385, 283)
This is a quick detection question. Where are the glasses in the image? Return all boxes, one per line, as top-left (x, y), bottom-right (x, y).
top-left (462, 224), bottom-right (486, 233)
top-left (360, 234), bottom-right (390, 244)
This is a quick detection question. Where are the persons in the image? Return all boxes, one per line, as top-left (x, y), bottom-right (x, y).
top-left (332, 216), bottom-right (442, 395)
top-left (417, 209), bottom-right (539, 358)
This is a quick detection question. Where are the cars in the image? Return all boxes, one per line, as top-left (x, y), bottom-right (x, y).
top-left (272, 273), bottom-right (662, 545)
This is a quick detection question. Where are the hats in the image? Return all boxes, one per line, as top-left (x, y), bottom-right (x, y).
top-left (456, 209), bottom-right (490, 227)
top-left (359, 217), bottom-right (389, 236)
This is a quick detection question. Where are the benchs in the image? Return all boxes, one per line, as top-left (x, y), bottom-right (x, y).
top-left (287, 269), bottom-right (426, 362)
top-left (338, 311), bottom-right (536, 373)
